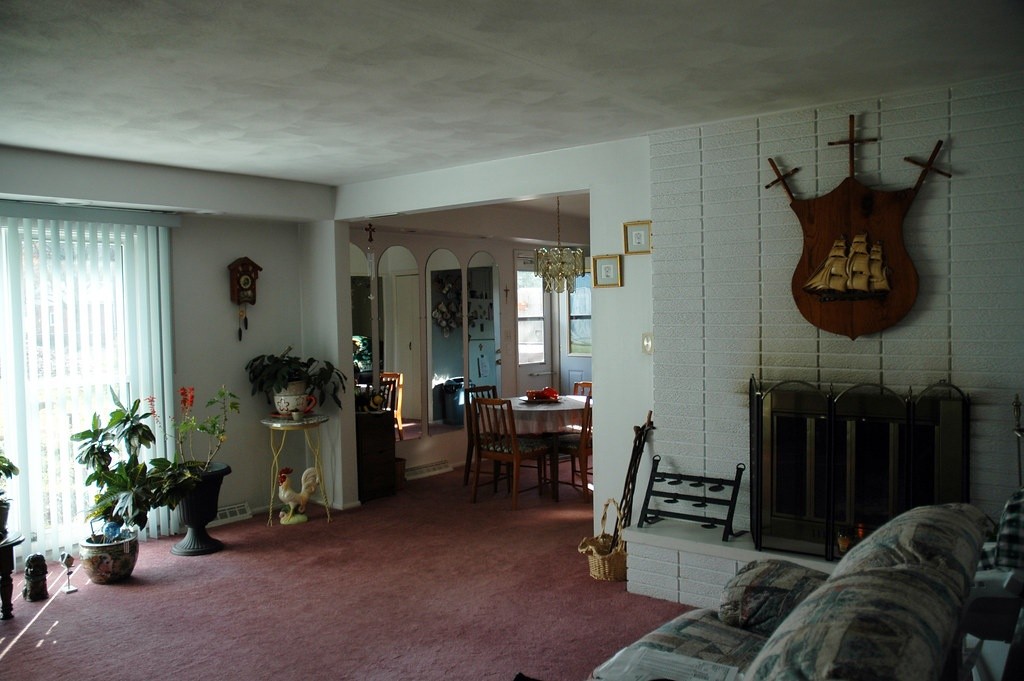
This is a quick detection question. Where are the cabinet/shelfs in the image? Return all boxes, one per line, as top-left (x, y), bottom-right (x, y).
top-left (356, 410), bottom-right (397, 504)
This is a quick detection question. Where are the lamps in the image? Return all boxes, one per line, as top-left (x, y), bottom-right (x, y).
top-left (533, 196), bottom-right (585, 294)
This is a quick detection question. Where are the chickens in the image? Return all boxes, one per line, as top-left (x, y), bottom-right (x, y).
top-left (278, 467), bottom-right (320, 523)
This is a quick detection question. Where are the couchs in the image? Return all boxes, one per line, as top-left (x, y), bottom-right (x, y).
top-left (586, 502), bottom-right (988, 681)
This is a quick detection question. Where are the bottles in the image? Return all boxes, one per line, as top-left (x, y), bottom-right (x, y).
top-left (853, 524), bottom-right (865, 546)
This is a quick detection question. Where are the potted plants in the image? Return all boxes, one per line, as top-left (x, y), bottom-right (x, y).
top-left (67, 383), bottom-right (204, 586)
top-left (0, 453), bottom-right (20, 540)
top-left (244, 346), bottom-right (348, 420)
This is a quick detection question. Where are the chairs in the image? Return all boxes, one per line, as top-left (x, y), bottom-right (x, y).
top-left (461, 387), bottom-right (512, 494)
top-left (380, 372), bottom-right (404, 441)
top-left (541, 383), bottom-right (593, 476)
top-left (547, 395), bottom-right (593, 504)
top-left (471, 396), bottom-right (556, 512)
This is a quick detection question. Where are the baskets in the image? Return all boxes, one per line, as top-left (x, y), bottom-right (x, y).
top-left (578, 498), bottom-right (627, 581)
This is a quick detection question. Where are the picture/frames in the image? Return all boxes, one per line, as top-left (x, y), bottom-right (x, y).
top-left (623, 220), bottom-right (653, 255)
top-left (591, 254), bottom-right (623, 288)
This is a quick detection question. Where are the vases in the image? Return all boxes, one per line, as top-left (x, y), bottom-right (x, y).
top-left (168, 461), bottom-right (233, 556)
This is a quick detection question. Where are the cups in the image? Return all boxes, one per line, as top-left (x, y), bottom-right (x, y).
top-left (838, 532), bottom-right (850, 555)
top-left (525, 390), bottom-right (558, 399)
top-left (291, 412), bottom-right (304, 420)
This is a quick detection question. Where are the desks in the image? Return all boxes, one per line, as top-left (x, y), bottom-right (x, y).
top-left (0, 534), bottom-right (25, 620)
top-left (260, 413), bottom-right (334, 528)
top-left (463, 395), bottom-right (593, 502)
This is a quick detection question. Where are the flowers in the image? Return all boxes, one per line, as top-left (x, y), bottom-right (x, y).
top-left (146, 384), bottom-right (242, 473)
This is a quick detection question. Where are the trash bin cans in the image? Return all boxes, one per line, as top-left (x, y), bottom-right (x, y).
top-left (443, 376), bottom-right (476, 425)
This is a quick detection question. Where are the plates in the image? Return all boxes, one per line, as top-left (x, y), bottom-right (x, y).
top-left (519, 396), bottom-right (562, 402)
